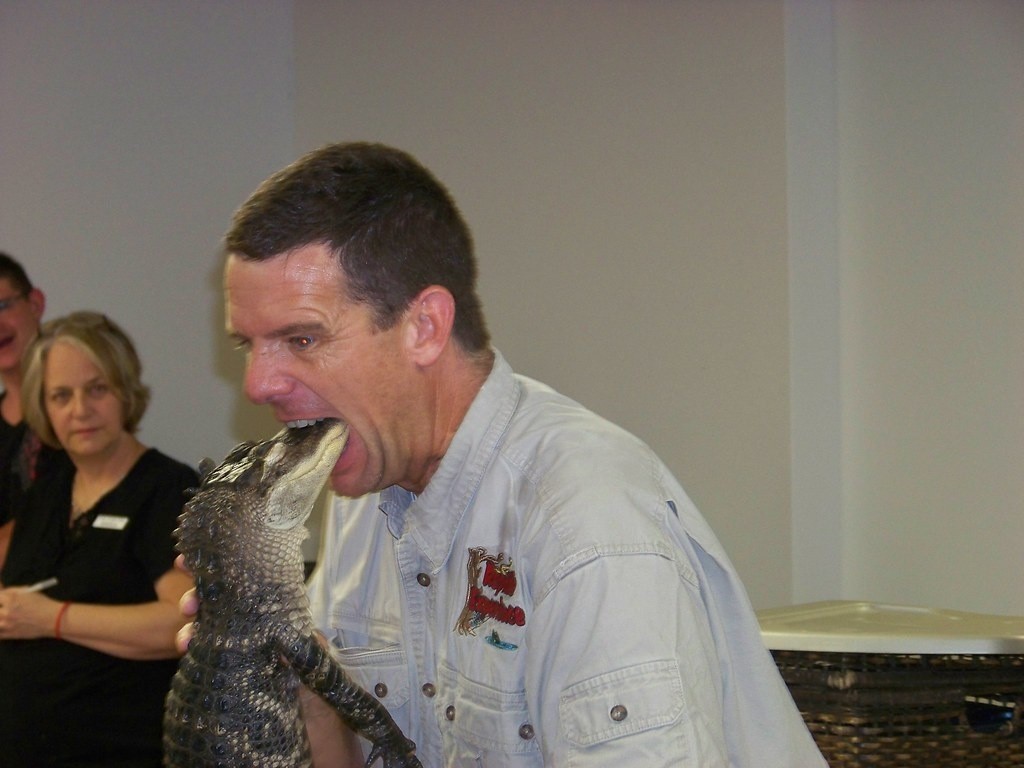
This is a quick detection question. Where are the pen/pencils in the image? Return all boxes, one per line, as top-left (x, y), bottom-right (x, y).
top-left (29, 579), bottom-right (57, 594)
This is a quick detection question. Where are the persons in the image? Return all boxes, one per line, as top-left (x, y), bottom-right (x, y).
top-left (0, 312), bottom-right (207, 768)
top-left (1, 252), bottom-right (50, 567)
top-left (217, 138), bottom-right (831, 768)
